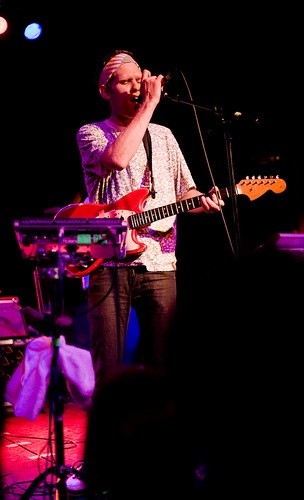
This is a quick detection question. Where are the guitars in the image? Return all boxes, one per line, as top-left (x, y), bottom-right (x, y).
top-left (51, 172), bottom-right (287, 279)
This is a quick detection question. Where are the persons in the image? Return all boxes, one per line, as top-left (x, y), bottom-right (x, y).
top-left (75, 52), bottom-right (226, 419)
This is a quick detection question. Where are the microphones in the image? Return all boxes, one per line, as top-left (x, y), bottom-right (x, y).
top-left (138, 73), bottom-right (172, 104)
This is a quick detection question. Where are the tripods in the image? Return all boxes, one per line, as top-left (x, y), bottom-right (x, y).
top-left (15, 218), bottom-right (126, 500)
